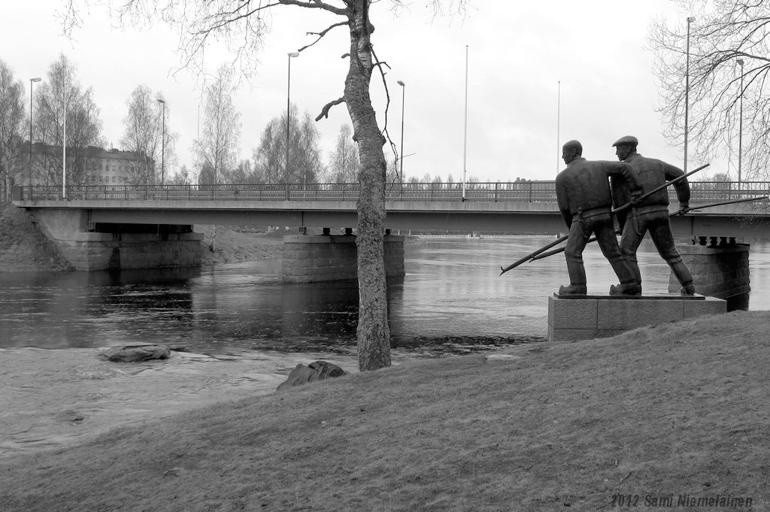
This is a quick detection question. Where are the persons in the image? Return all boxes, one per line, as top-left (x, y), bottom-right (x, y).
top-left (610, 135), bottom-right (697, 295)
top-left (553, 139), bottom-right (644, 297)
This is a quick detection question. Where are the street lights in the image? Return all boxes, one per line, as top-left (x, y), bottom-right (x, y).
top-left (736, 59), bottom-right (744, 191)
top-left (29, 77), bottom-right (42, 200)
top-left (157, 99), bottom-right (165, 188)
top-left (397, 80), bottom-right (405, 199)
top-left (683, 17), bottom-right (696, 174)
top-left (286, 52), bottom-right (299, 200)
